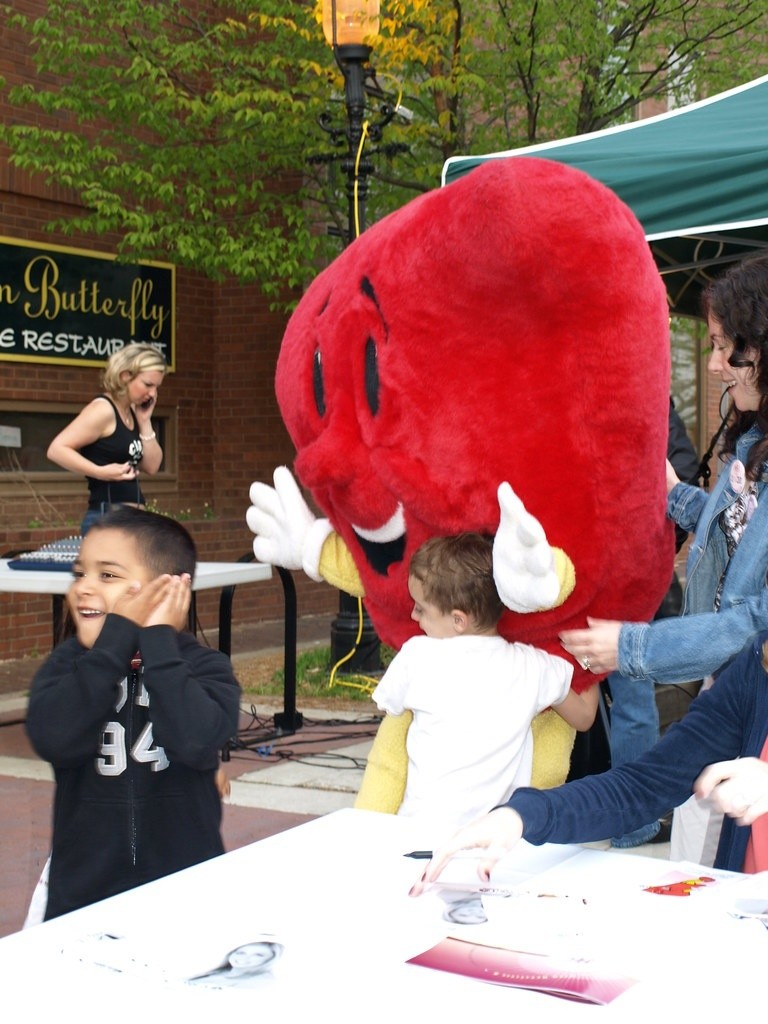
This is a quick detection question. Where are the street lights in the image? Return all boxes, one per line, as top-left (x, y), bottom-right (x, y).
top-left (305, 0), bottom-right (412, 674)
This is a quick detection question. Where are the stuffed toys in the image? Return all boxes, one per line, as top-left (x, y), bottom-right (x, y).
top-left (245, 156), bottom-right (676, 816)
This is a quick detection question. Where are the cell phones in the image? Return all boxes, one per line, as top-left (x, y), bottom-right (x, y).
top-left (141, 400), bottom-right (154, 409)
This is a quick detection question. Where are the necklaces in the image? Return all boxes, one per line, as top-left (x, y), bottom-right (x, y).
top-left (113, 394), bottom-right (131, 425)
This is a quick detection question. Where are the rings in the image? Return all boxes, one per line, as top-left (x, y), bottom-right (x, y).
top-left (580, 656), bottom-right (590, 672)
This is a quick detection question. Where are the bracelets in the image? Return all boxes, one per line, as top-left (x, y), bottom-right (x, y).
top-left (140, 431), bottom-right (156, 441)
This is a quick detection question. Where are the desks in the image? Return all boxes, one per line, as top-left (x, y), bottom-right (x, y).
top-left (0, 560), bottom-right (273, 763)
top-left (0, 807), bottom-right (768, 1024)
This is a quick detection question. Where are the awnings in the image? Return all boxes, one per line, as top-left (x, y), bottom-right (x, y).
top-left (440, 75), bottom-right (768, 320)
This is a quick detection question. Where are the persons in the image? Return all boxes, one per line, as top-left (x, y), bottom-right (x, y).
top-left (558, 254), bottom-right (768, 869)
top-left (46, 343), bottom-right (167, 537)
top-left (368, 532), bottom-right (600, 830)
top-left (408, 630), bottom-right (768, 897)
top-left (437, 889), bottom-right (488, 925)
top-left (188, 941), bottom-right (285, 1001)
top-left (598, 396), bottom-right (700, 849)
top-left (24, 504), bottom-right (243, 922)
